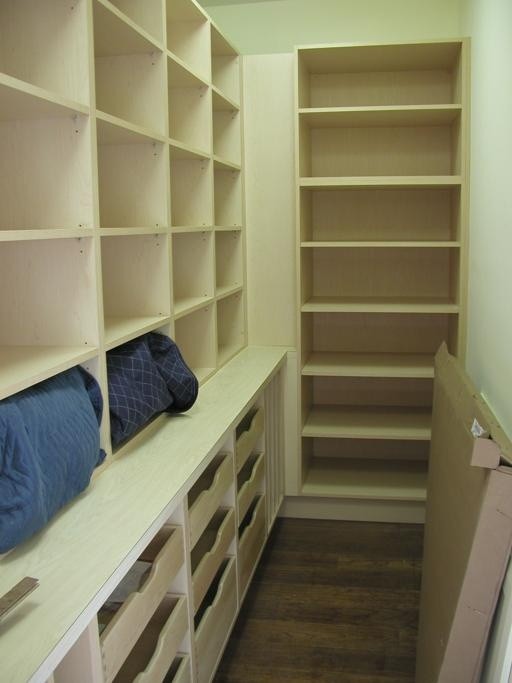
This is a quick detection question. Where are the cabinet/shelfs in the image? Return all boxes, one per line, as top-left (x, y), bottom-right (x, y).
top-left (292, 35), bottom-right (472, 504)
top-left (0, 1), bottom-right (289, 682)
